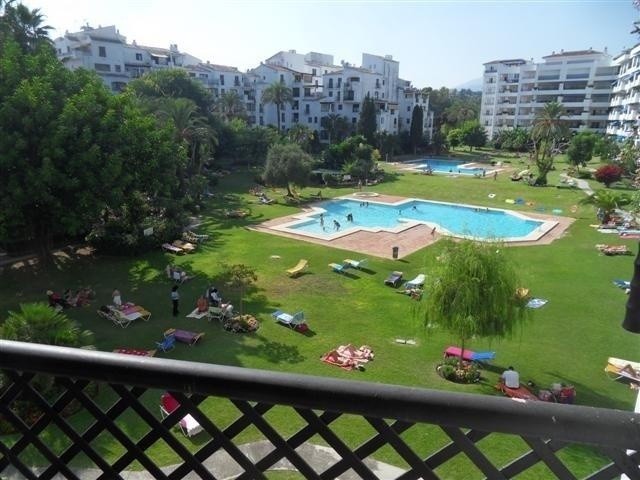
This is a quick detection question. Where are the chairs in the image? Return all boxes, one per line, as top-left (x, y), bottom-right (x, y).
top-left (604, 356), bottom-right (640, 385)
top-left (164, 328), bottom-right (206, 345)
top-left (272, 310), bottom-right (305, 329)
top-left (96, 301), bottom-right (151, 330)
top-left (443, 345), bottom-right (495, 370)
top-left (286, 258), bottom-right (427, 296)
top-left (161, 239), bottom-right (198, 256)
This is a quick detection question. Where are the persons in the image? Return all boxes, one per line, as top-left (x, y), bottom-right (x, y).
top-left (558, 382), bottom-right (569, 404)
top-left (324, 343), bottom-right (375, 368)
top-left (171, 285), bottom-right (180, 317)
top-left (624, 210), bottom-right (636, 229)
top-left (359, 201), bottom-right (369, 207)
top-left (319, 212), bottom-right (353, 232)
top-left (111, 287), bottom-right (121, 311)
top-left (358, 179), bottom-right (362, 193)
top-left (616, 364), bottom-right (640, 379)
top-left (196, 280), bottom-right (234, 321)
top-left (501, 366), bottom-right (520, 389)
top-left (483, 169), bottom-right (485, 176)
top-left (398, 289), bottom-right (423, 295)
top-left (318, 190), bottom-right (322, 198)
top-left (601, 211), bottom-right (611, 225)
top-left (474, 207), bottom-right (489, 212)
top-left (411, 205), bottom-right (417, 213)
top-left (430, 227), bottom-right (436, 238)
top-left (398, 210), bottom-right (401, 215)
top-left (492, 172), bottom-right (498, 181)
top-left (45, 289), bottom-right (82, 309)
